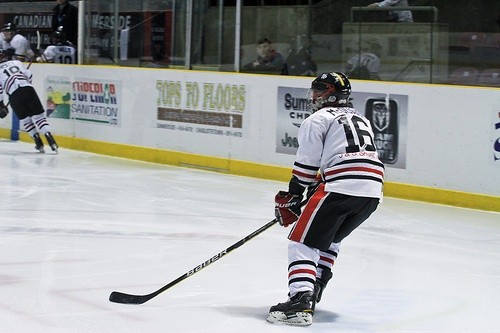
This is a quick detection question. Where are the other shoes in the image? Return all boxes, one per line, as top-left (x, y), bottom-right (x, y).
top-left (266, 280), bottom-right (324, 326)
top-left (33, 132), bottom-right (45, 153)
top-left (44, 131), bottom-right (59, 155)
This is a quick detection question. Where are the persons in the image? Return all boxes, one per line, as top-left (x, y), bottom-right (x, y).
top-left (241, 34), bottom-right (330, 75)
top-left (344, 44), bottom-right (384, 80)
top-left (267, 72), bottom-right (383, 327)
top-left (0, 0), bottom-right (79, 155)
top-left (367, 0), bottom-right (414, 23)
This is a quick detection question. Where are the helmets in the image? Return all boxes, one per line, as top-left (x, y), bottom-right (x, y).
top-left (0, 41), bottom-right (16, 60)
top-left (345, 50), bottom-right (379, 78)
top-left (1, 23), bottom-right (17, 42)
top-left (307, 71), bottom-right (352, 114)
top-left (53, 26), bottom-right (69, 39)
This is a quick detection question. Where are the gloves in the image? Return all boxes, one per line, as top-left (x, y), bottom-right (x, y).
top-left (0, 100), bottom-right (9, 119)
top-left (274, 190), bottom-right (304, 227)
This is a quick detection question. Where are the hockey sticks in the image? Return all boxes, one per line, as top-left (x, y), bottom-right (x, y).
top-left (5, 43), bottom-right (44, 108)
top-left (109, 198), bottom-right (309, 305)
